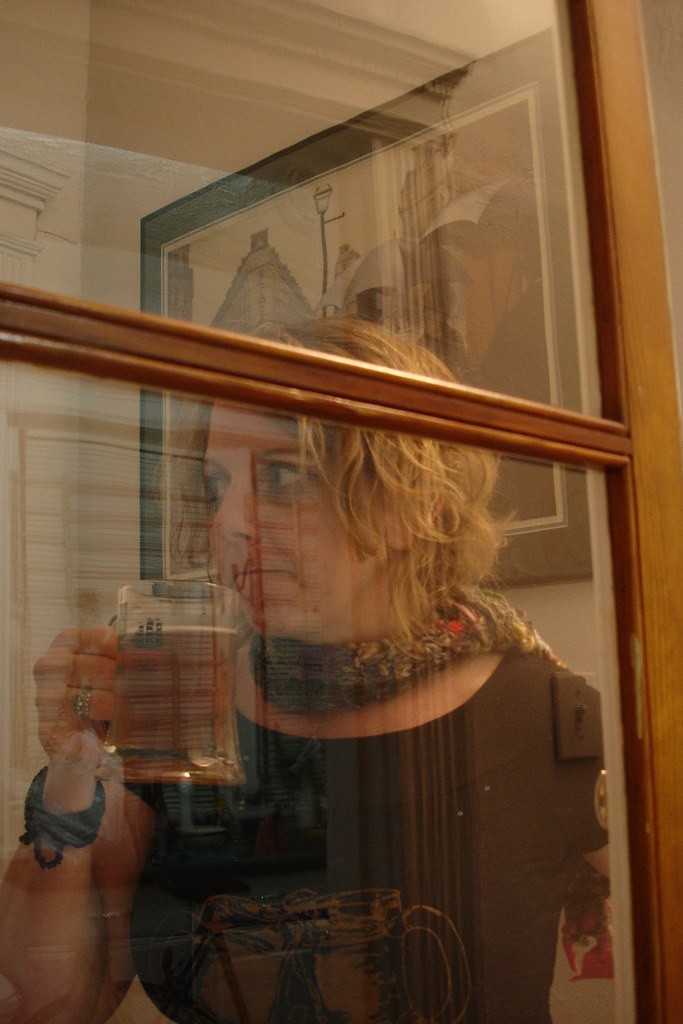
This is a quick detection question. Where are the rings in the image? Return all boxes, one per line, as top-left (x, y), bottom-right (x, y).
top-left (73, 686), bottom-right (94, 721)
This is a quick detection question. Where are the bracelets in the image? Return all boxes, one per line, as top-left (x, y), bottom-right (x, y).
top-left (19, 767), bottom-right (106, 870)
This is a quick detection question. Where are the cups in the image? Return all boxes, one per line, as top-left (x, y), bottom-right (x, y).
top-left (83, 579), bottom-right (250, 788)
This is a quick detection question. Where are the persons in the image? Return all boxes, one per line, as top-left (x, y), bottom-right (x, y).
top-left (0, 317), bottom-right (605, 1024)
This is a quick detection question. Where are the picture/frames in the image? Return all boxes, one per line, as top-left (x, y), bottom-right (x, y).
top-left (136, 22), bottom-right (593, 593)
